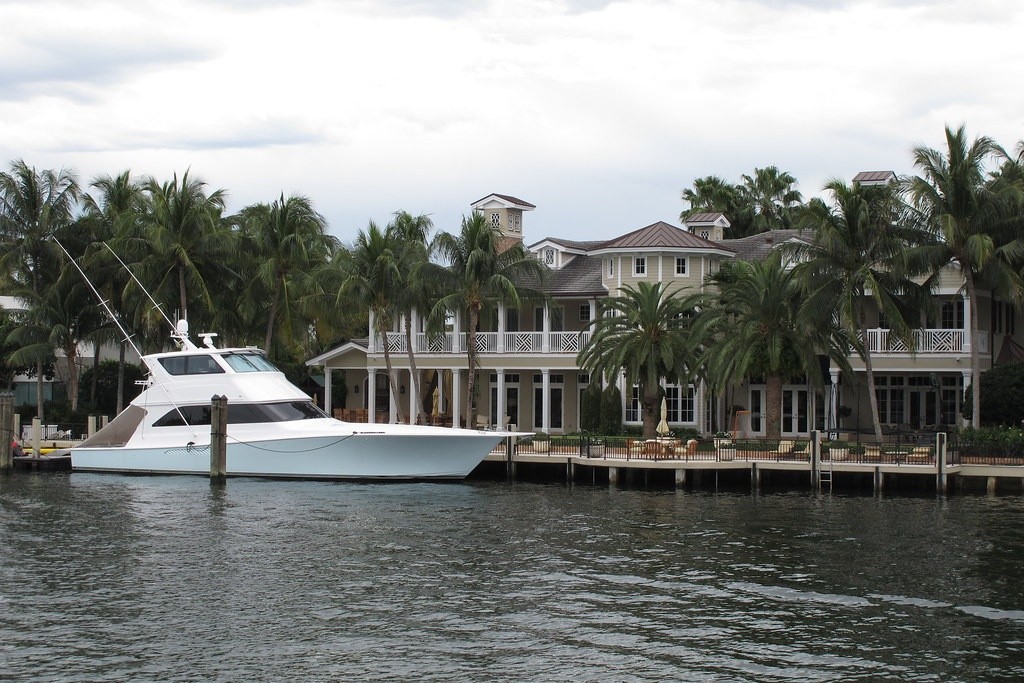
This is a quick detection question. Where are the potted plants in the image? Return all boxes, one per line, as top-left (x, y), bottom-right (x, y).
top-left (531, 432), bottom-right (552, 452)
top-left (947, 434), bottom-right (960, 464)
top-left (829, 439), bottom-right (850, 461)
top-left (583, 429), bottom-right (608, 458)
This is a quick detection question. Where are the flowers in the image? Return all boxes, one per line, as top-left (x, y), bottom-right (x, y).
top-left (658, 431), bottom-right (675, 437)
top-left (714, 431), bottom-right (732, 438)
top-left (720, 443), bottom-right (736, 449)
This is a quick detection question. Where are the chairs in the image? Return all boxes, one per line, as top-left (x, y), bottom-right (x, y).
top-left (51, 429), bottom-right (72, 440)
top-left (769, 440), bottom-right (795, 460)
top-left (334, 409), bottom-right (367, 423)
top-left (794, 441), bottom-right (823, 461)
top-left (625, 439), bottom-right (699, 459)
top-left (417, 413), bottom-right (453, 427)
top-left (864, 445), bottom-right (882, 463)
top-left (906, 447), bottom-right (931, 465)
top-left (476, 414), bottom-right (511, 431)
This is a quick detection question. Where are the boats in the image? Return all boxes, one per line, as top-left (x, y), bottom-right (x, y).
top-left (51, 235), bottom-right (536, 483)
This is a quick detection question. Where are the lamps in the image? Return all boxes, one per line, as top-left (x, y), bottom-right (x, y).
top-left (401, 385), bottom-right (406, 394)
top-left (425, 378), bottom-right (430, 390)
top-left (354, 384), bottom-right (359, 393)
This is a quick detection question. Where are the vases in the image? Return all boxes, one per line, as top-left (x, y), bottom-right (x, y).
top-left (656, 437), bottom-right (675, 439)
top-left (720, 449), bottom-right (737, 461)
top-left (714, 438), bottom-right (732, 449)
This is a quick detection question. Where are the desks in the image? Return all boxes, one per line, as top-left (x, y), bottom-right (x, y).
top-left (885, 451), bottom-right (908, 464)
